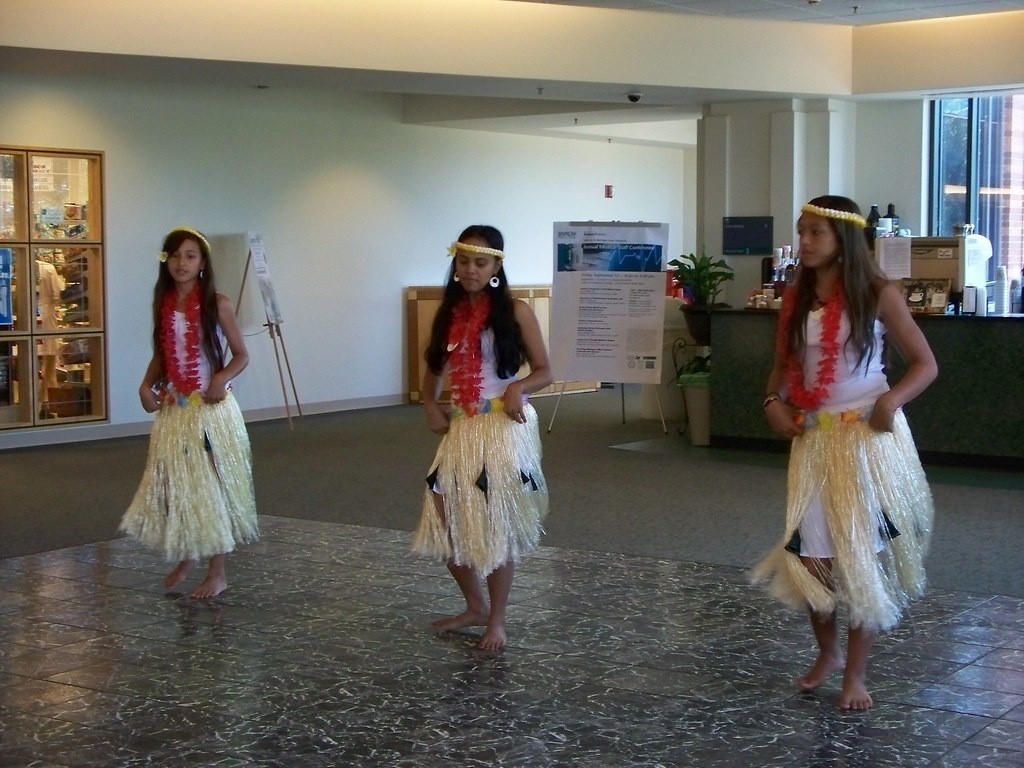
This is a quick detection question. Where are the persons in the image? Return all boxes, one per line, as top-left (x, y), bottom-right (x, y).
top-left (119, 228), bottom-right (262, 599)
top-left (37, 257), bottom-right (60, 419)
top-left (745, 196), bottom-right (940, 710)
top-left (412, 225), bottom-right (554, 652)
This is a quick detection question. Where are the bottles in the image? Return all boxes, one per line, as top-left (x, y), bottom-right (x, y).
top-left (866, 206), bottom-right (882, 239)
top-left (771, 246), bottom-right (791, 283)
top-left (883, 204), bottom-right (899, 237)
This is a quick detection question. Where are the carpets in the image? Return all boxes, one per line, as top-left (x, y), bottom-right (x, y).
top-left (0, 515), bottom-right (1024, 768)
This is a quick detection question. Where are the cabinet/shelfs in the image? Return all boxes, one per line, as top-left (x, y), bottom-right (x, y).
top-left (57, 219), bottom-right (91, 415)
top-left (668, 337), bottom-right (711, 437)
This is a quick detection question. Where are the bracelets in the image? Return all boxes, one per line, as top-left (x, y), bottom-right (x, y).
top-left (762, 392), bottom-right (781, 408)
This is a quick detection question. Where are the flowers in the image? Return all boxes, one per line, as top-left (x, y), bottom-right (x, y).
top-left (158, 251), bottom-right (168, 262)
top-left (446, 244), bottom-right (457, 258)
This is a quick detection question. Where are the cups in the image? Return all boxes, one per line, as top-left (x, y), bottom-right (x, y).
top-left (995, 266), bottom-right (1021, 313)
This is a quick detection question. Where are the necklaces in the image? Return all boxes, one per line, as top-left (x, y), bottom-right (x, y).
top-left (161, 278), bottom-right (201, 396)
top-left (448, 294), bottom-right (489, 406)
top-left (776, 269), bottom-right (844, 413)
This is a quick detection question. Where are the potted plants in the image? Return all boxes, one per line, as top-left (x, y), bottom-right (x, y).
top-left (667, 242), bottom-right (737, 345)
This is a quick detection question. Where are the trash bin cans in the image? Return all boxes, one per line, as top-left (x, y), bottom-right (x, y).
top-left (680, 374), bottom-right (710, 446)
